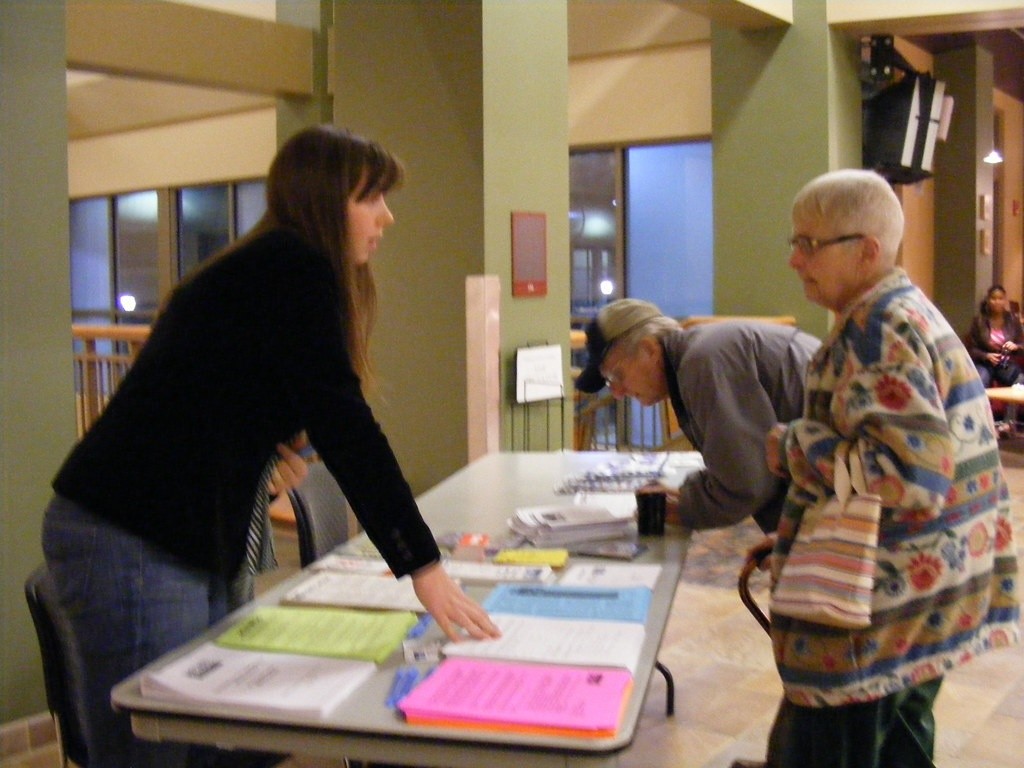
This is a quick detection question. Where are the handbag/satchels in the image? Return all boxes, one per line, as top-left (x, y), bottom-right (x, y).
top-left (768, 437), bottom-right (882, 630)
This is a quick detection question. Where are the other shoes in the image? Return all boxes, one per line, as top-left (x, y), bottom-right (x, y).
top-left (731, 758), bottom-right (767, 768)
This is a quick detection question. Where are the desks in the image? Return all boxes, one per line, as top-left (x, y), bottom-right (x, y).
top-left (108, 450), bottom-right (705, 768)
top-left (984, 386), bottom-right (1024, 440)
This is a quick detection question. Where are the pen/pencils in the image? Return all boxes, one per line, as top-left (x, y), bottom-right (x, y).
top-left (386, 666), bottom-right (434, 708)
top-left (651, 452), bottom-right (672, 483)
top-left (510, 587), bottom-right (620, 600)
top-left (411, 612), bottom-right (432, 637)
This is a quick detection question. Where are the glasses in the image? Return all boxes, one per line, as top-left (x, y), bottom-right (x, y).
top-left (787, 234), bottom-right (865, 255)
top-left (606, 351), bottom-right (635, 388)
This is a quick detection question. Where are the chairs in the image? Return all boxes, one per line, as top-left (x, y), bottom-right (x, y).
top-left (24, 563), bottom-right (67, 768)
top-left (286, 462), bottom-right (348, 570)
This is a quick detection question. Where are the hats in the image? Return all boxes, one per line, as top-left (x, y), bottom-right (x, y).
top-left (574, 299), bottom-right (661, 392)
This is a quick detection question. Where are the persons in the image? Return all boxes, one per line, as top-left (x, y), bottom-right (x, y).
top-left (42, 126), bottom-right (502, 768)
top-left (576, 300), bottom-right (824, 537)
top-left (731, 169), bottom-right (1018, 767)
top-left (964, 285), bottom-right (1024, 426)
